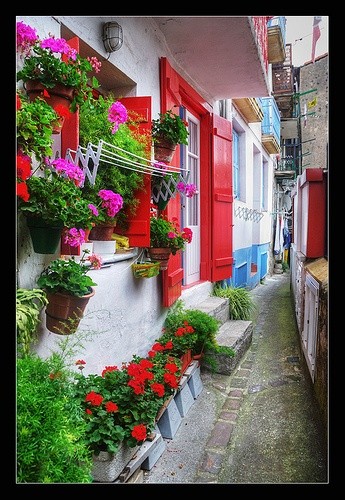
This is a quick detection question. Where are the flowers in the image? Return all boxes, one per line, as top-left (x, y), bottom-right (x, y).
top-left (16, 21), bottom-right (197, 247)
top-left (36, 248), bottom-right (104, 298)
top-left (149, 211), bottom-right (193, 255)
top-left (48, 320), bottom-right (199, 459)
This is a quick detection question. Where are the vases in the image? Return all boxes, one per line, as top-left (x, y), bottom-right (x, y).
top-left (44, 285), bottom-right (95, 335)
top-left (148, 247), bottom-right (171, 270)
top-left (26, 79), bottom-right (76, 134)
top-left (154, 137), bottom-right (178, 164)
top-left (60, 223), bottom-right (80, 255)
top-left (177, 348), bottom-right (194, 382)
top-left (90, 218), bottom-right (116, 241)
top-left (132, 260), bottom-right (161, 279)
top-left (23, 211), bottom-right (66, 255)
top-left (82, 222), bottom-right (95, 241)
top-left (89, 422), bottom-right (148, 483)
top-left (153, 187), bottom-right (173, 212)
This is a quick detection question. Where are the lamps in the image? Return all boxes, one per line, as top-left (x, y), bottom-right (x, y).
top-left (102, 20), bottom-right (123, 53)
